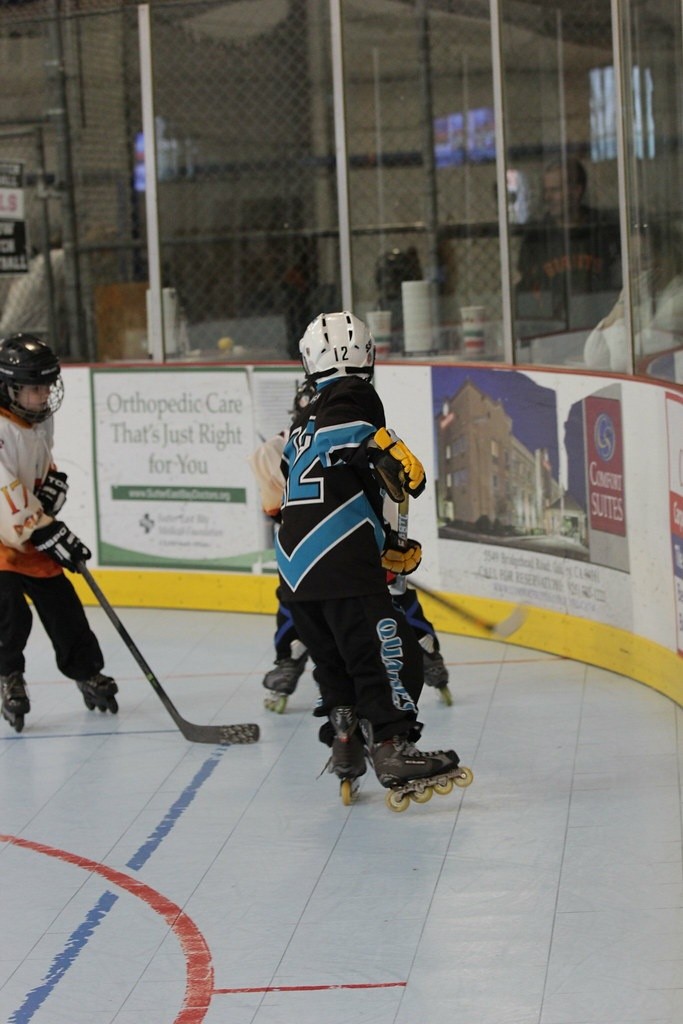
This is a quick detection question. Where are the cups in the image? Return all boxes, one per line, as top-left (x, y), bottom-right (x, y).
top-left (461, 306), bottom-right (487, 356)
top-left (366, 312), bottom-right (392, 361)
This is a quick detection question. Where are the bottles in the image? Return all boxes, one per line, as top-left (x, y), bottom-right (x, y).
top-left (178, 306), bottom-right (191, 357)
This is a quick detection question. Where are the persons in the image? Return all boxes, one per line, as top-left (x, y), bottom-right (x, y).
top-left (518, 159), bottom-right (635, 293)
top-left (0, 223), bottom-right (124, 362)
top-left (147, 234), bottom-right (223, 323)
top-left (244, 142), bottom-right (319, 360)
top-left (1, 331), bottom-right (119, 732)
top-left (160, 146), bottom-right (240, 268)
top-left (251, 311), bottom-right (473, 813)
top-left (582, 220), bottom-right (683, 371)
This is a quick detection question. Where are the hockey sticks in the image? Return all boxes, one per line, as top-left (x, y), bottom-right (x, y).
top-left (406, 578), bottom-right (527, 638)
top-left (79, 561), bottom-right (259, 743)
top-left (387, 471), bottom-right (410, 596)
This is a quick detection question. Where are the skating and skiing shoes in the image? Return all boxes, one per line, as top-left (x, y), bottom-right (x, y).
top-left (328, 706), bottom-right (371, 804)
top-left (0, 672), bottom-right (31, 733)
top-left (262, 640), bottom-right (308, 714)
top-left (75, 672), bottom-right (120, 715)
top-left (358, 719), bottom-right (474, 813)
top-left (418, 631), bottom-right (452, 706)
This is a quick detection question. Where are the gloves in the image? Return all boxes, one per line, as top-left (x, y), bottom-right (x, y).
top-left (37, 469), bottom-right (69, 517)
top-left (30, 520), bottom-right (92, 575)
top-left (379, 529), bottom-right (422, 576)
top-left (367, 427), bottom-right (426, 504)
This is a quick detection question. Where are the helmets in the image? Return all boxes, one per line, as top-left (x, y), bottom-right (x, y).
top-left (297, 311), bottom-right (376, 386)
top-left (0, 333), bottom-right (65, 422)
